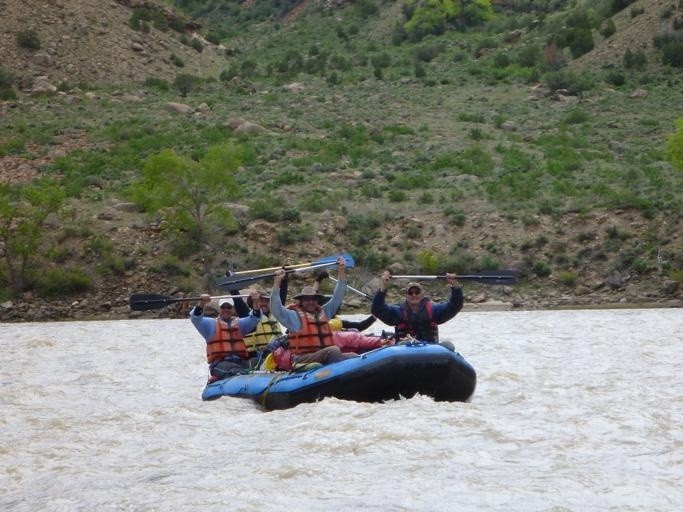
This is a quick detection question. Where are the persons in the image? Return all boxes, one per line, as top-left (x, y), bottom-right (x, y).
top-left (371, 271), bottom-right (464, 345)
top-left (313, 271), bottom-right (382, 350)
top-left (190, 291), bottom-right (262, 384)
top-left (270, 256), bottom-right (360, 365)
top-left (226, 264), bottom-right (288, 366)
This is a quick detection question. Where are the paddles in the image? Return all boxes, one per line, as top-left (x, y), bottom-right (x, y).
top-left (388, 270), bottom-right (515, 286)
top-left (216, 254), bottom-right (354, 290)
top-left (130, 294), bottom-right (251, 311)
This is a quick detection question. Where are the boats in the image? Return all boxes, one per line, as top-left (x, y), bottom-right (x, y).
top-left (199, 338), bottom-right (478, 411)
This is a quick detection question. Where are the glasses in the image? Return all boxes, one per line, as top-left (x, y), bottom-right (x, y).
top-left (408, 291), bottom-right (421, 296)
top-left (221, 306), bottom-right (232, 310)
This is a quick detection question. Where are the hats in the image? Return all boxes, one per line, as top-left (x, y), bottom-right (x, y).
top-left (406, 282), bottom-right (423, 292)
top-left (247, 289), bottom-right (273, 309)
top-left (218, 295), bottom-right (235, 307)
top-left (293, 287), bottom-right (326, 301)
